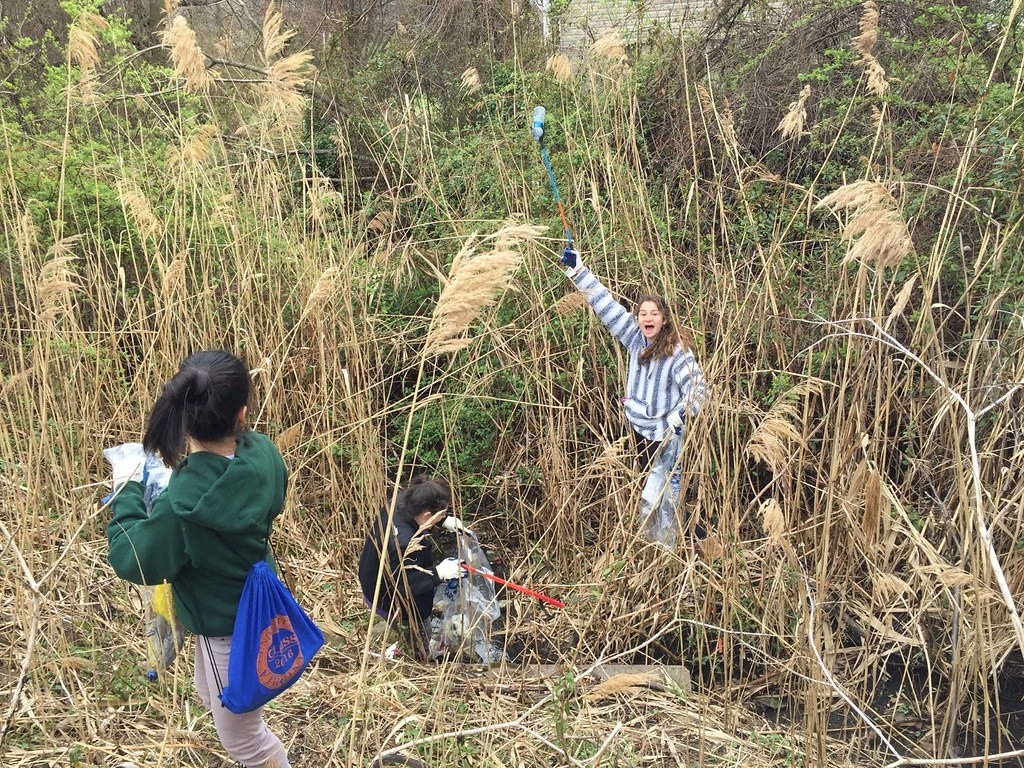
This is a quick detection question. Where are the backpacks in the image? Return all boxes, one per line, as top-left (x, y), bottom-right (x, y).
top-left (193, 509), bottom-right (325, 715)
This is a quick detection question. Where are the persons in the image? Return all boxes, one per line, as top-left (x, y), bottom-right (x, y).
top-left (105, 350), bottom-right (293, 768)
top-left (561, 245), bottom-right (709, 551)
top-left (358, 476), bottom-right (469, 661)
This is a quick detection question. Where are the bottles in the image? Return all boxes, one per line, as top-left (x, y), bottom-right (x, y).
top-left (147, 629), bottom-right (184, 680)
top-left (532, 106), bottom-right (545, 140)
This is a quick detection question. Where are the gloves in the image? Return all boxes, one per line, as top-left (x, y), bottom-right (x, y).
top-left (666, 407), bottom-right (686, 436)
top-left (560, 247), bottom-right (583, 272)
top-left (441, 516), bottom-right (471, 537)
top-left (436, 557), bottom-right (469, 581)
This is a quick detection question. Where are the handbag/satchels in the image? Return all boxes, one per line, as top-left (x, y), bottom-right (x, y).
top-left (146, 617), bottom-right (186, 670)
